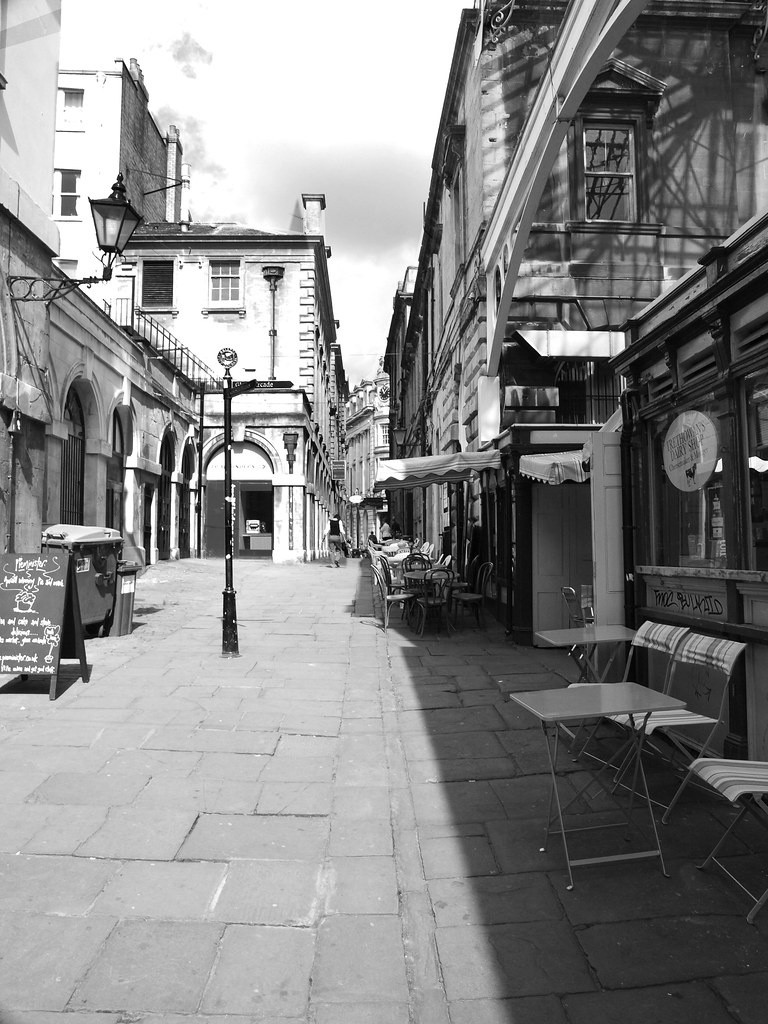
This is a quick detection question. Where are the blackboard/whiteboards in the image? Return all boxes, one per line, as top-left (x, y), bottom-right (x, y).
top-left (1, 552), bottom-right (71, 675)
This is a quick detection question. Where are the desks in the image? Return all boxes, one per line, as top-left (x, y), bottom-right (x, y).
top-left (404, 572), bottom-right (460, 589)
top-left (510, 682), bottom-right (687, 890)
top-left (534, 626), bottom-right (637, 754)
top-left (407, 564), bottom-right (446, 570)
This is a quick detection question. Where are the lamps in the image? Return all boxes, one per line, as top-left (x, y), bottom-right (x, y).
top-left (8, 171), bottom-right (145, 306)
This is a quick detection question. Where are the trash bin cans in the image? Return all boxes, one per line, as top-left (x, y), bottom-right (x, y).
top-left (87, 557), bottom-right (143, 638)
top-left (41, 522), bottom-right (124, 638)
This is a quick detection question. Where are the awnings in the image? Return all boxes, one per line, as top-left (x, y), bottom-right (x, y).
top-left (518, 450), bottom-right (591, 486)
top-left (517, 329), bottom-right (626, 358)
top-left (581, 407), bottom-right (623, 473)
top-left (373, 449), bottom-right (502, 492)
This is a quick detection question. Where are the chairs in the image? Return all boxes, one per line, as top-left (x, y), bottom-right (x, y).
top-left (688, 759), bottom-right (768, 924)
top-left (562, 585), bottom-right (595, 661)
top-left (368, 538), bottom-right (493, 639)
top-left (573, 621), bottom-right (747, 826)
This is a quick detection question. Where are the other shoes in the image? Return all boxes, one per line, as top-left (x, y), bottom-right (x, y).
top-left (335, 562), bottom-right (339, 567)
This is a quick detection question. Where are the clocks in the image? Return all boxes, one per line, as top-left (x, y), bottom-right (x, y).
top-left (379, 385), bottom-right (390, 402)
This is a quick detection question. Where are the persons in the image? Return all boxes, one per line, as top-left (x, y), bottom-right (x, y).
top-left (368, 531), bottom-right (377, 546)
top-left (380, 518), bottom-right (392, 540)
top-left (390, 516), bottom-right (402, 536)
top-left (322, 513), bottom-right (346, 568)
